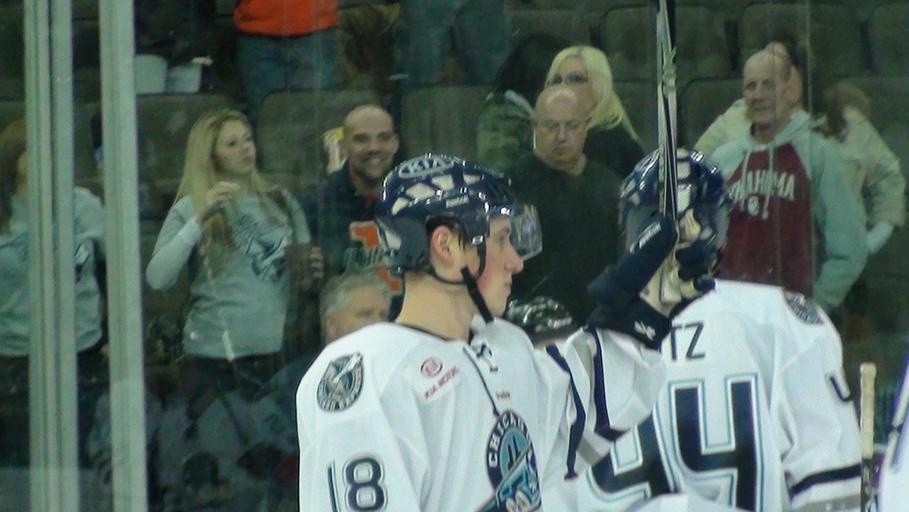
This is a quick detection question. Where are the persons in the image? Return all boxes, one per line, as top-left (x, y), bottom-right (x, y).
top-left (575, 149), bottom-right (862, 511)
top-left (147, 108), bottom-right (325, 399)
top-left (501, 31), bottom-right (573, 102)
top-left (96, 359), bottom-right (291, 506)
top-left (541, 46), bottom-right (646, 177)
top-left (492, 85), bottom-right (620, 266)
top-left (413, 93), bottom-right (520, 139)
top-left (695, 35), bottom-right (905, 256)
top-left (296, 153), bottom-right (715, 511)
top-left (606, 17), bottom-right (688, 65)
top-left (355, 8), bottom-right (499, 84)
top-left (804, 11), bottom-right (903, 55)
top-left (711, 49), bottom-right (868, 313)
top-left (253, 271), bottom-right (392, 408)
top-left (2, 117), bottom-right (106, 465)
top-left (232, 1), bottom-right (341, 104)
top-left (305, 106), bottom-right (401, 274)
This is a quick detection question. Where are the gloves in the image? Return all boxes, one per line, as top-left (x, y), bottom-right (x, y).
top-left (592, 219), bottom-right (714, 328)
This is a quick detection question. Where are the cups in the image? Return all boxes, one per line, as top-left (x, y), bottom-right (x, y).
top-left (286, 242), bottom-right (316, 293)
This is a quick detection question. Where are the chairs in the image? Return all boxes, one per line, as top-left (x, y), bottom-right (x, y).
top-left (258, 90), bottom-right (384, 199)
top-left (830, 75), bottom-right (909, 136)
top-left (610, 78), bottom-right (659, 159)
top-left (0, 101), bottom-right (99, 186)
top-left (738, 1), bottom-right (866, 81)
top-left (399, 84), bottom-right (487, 163)
top-left (506, 8), bottom-right (590, 53)
top-left (139, 219), bottom-right (191, 317)
top-left (133, 91), bottom-right (234, 198)
top-left (600, 5), bottom-right (732, 88)
top-left (868, 1), bottom-right (909, 75)
top-left (678, 76), bottom-right (747, 149)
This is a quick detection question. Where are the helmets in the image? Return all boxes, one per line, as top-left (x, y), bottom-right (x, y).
top-left (620, 150), bottom-right (731, 249)
top-left (374, 158), bottom-right (514, 276)
